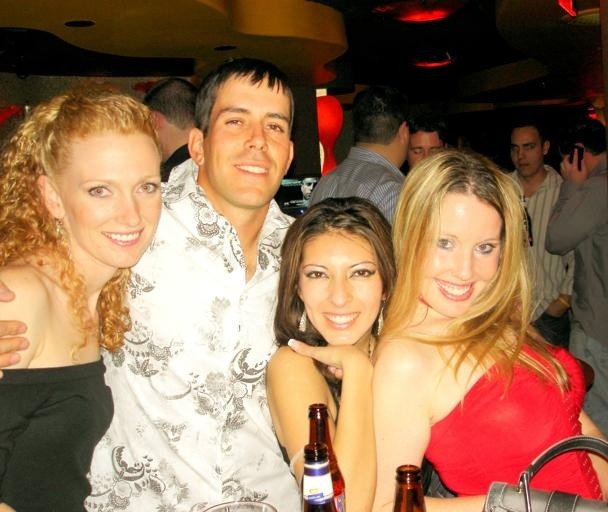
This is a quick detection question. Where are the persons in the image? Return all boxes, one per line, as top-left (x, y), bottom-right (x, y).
top-left (1, 79), bottom-right (165, 511)
top-left (139, 75), bottom-right (199, 184)
top-left (306, 85), bottom-right (605, 444)
top-left (264, 196), bottom-right (397, 512)
top-left (0, 55), bottom-right (302, 510)
top-left (371, 148), bottom-right (607, 510)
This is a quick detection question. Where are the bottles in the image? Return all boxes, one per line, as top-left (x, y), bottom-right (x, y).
top-left (301, 440), bottom-right (341, 512)
top-left (302, 403), bottom-right (344, 512)
top-left (392, 464), bottom-right (426, 512)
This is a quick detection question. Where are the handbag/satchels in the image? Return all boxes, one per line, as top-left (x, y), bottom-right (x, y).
top-left (483, 436), bottom-right (608, 512)
